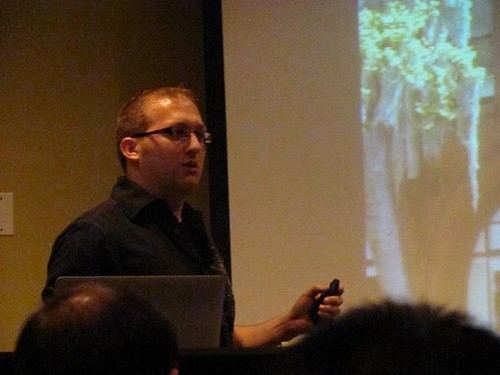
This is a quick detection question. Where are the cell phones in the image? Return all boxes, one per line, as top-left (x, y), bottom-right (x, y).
top-left (307, 279), bottom-right (339, 325)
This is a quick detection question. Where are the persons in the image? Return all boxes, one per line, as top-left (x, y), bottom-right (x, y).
top-left (45, 85), bottom-right (344, 373)
top-left (284, 302), bottom-right (497, 373)
top-left (15, 283), bottom-right (178, 375)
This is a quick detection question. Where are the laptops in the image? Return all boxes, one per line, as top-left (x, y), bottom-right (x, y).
top-left (55, 275), bottom-right (225, 350)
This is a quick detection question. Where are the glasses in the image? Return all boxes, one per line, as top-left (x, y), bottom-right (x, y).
top-left (127, 124), bottom-right (213, 142)
